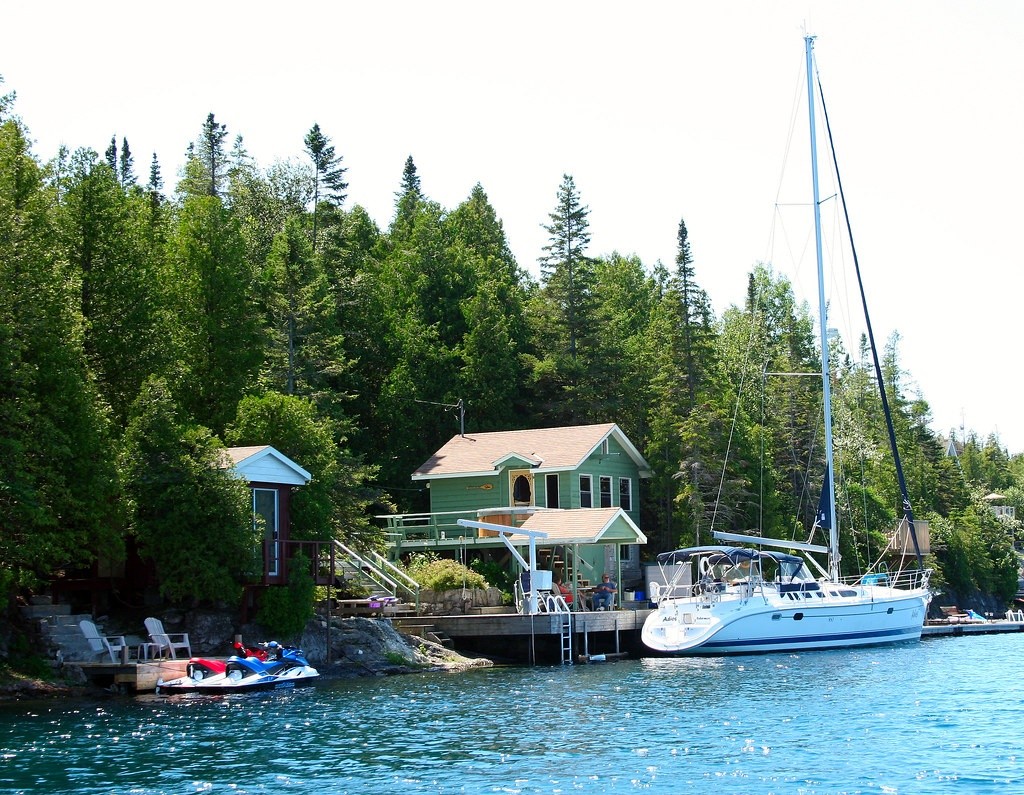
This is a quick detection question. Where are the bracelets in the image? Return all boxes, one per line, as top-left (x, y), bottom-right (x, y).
top-left (608, 588), bottom-right (610, 591)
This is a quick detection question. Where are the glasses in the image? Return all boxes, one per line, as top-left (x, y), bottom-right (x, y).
top-left (604, 577), bottom-right (610, 579)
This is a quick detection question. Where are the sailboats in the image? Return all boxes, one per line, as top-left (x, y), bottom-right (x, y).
top-left (638, 34), bottom-right (935, 657)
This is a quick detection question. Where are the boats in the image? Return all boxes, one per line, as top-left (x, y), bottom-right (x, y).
top-left (156, 641), bottom-right (322, 694)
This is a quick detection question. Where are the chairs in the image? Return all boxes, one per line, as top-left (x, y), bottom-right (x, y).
top-left (80, 620), bottom-right (126, 663)
top-left (144, 617), bottom-right (192, 661)
top-left (515, 572), bottom-right (617, 612)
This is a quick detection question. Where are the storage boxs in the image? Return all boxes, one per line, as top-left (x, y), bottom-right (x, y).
top-left (624, 590), bottom-right (645, 601)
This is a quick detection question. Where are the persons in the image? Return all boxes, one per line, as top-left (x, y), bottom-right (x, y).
top-left (551, 577), bottom-right (591, 612)
top-left (591, 574), bottom-right (618, 611)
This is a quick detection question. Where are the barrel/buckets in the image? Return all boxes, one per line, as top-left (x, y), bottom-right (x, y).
top-left (624, 590), bottom-right (635, 601)
top-left (635, 591), bottom-right (645, 601)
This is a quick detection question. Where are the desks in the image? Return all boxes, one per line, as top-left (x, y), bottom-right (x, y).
top-left (337, 599), bottom-right (398, 617)
top-left (137, 642), bottom-right (162, 661)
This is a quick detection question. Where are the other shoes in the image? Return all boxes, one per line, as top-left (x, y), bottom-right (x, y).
top-left (596, 608), bottom-right (599, 611)
top-left (599, 606), bottom-right (605, 611)
